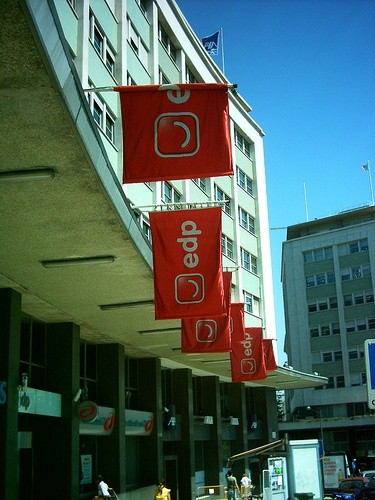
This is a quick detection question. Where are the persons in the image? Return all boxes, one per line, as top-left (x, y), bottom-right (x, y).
top-left (94, 474), bottom-right (111, 500)
top-left (337, 469), bottom-right (344, 486)
top-left (240, 472), bottom-right (252, 485)
top-left (154, 479), bottom-right (171, 500)
top-left (226, 470), bottom-right (240, 500)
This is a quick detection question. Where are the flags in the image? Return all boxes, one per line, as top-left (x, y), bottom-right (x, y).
top-left (263, 339), bottom-right (277, 371)
top-left (181, 271), bottom-right (232, 354)
top-left (230, 303), bottom-right (245, 341)
top-left (202, 31), bottom-right (218, 55)
top-left (114, 84), bottom-right (234, 184)
top-left (362, 164), bottom-right (368, 170)
top-left (230, 327), bottom-right (267, 382)
top-left (149, 206), bottom-right (227, 321)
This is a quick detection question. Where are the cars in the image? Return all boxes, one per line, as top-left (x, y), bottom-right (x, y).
top-left (338, 476), bottom-right (369, 500)
top-left (360, 470), bottom-right (375, 498)
